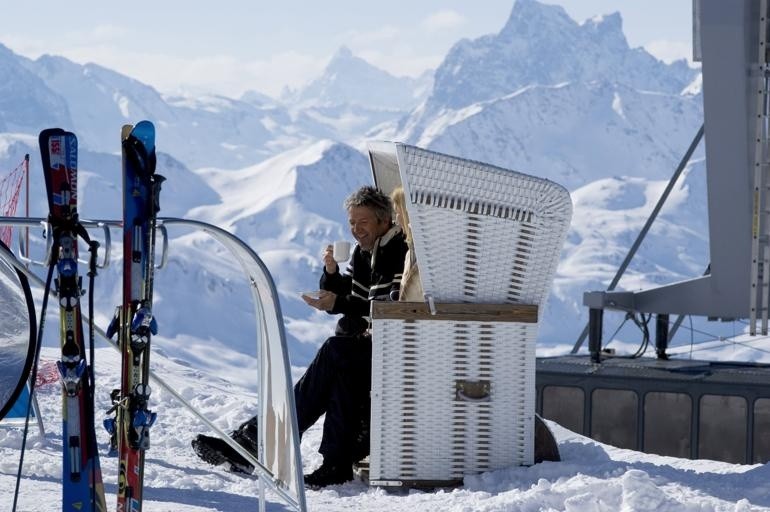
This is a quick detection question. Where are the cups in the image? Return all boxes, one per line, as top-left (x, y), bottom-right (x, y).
top-left (332, 241), bottom-right (351, 263)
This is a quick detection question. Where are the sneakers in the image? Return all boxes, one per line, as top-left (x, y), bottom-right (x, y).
top-left (192, 431), bottom-right (257, 474)
top-left (304, 458), bottom-right (353, 488)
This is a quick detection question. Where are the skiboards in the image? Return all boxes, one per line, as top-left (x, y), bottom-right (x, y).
top-left (105, 121), bottom-right (166, 512)
top-left (38, 127), bottom-right (106, 511)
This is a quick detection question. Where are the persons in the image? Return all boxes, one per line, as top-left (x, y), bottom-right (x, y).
top-left (191, 188), bottom-right (424, 493)
top-left (298, 184), bottom-right (410, 463)
